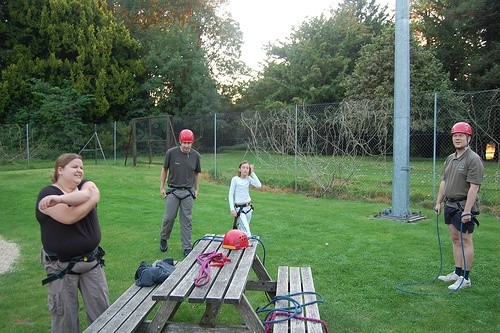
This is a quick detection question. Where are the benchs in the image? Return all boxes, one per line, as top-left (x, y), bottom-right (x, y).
top-left (273, 265), bottom-right (323, 333)
top-left (81, 260), bottom-right (178, 333)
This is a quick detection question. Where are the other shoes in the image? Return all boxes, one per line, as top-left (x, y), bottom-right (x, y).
top-left (183, 248), bottom-right (191, 256)
top-left (160, 239), bottom-right (167, 253)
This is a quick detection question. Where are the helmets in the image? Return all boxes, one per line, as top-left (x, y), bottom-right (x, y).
top-left (222, 229), bottom-right (249, 251)
top-left (178, 130), bottom-right (195, 144)
top-left (451, 122), bottom-right (474, 137)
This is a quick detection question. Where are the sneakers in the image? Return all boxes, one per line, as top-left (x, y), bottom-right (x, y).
top-left (438, 271), bottom-right (461, 283)
top-left (449, 274), bottom-right (471, 291)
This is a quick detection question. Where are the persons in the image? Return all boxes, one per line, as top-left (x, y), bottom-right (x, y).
top-left (228, 160), bottom-right (262, 238)
top-left (433, 122), bottom-right (483, 289)
top-left (35, 153), bottom-right (111, 333)
top-left (137, 143), bottom-right (143, 153)
top-left (159, 128), bottom-right (201, 257)
top-left (479, 143), bottom-right (499, 162)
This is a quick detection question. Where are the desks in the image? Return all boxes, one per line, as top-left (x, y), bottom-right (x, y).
top-left (147, 234), bottom-right (273, 333)
